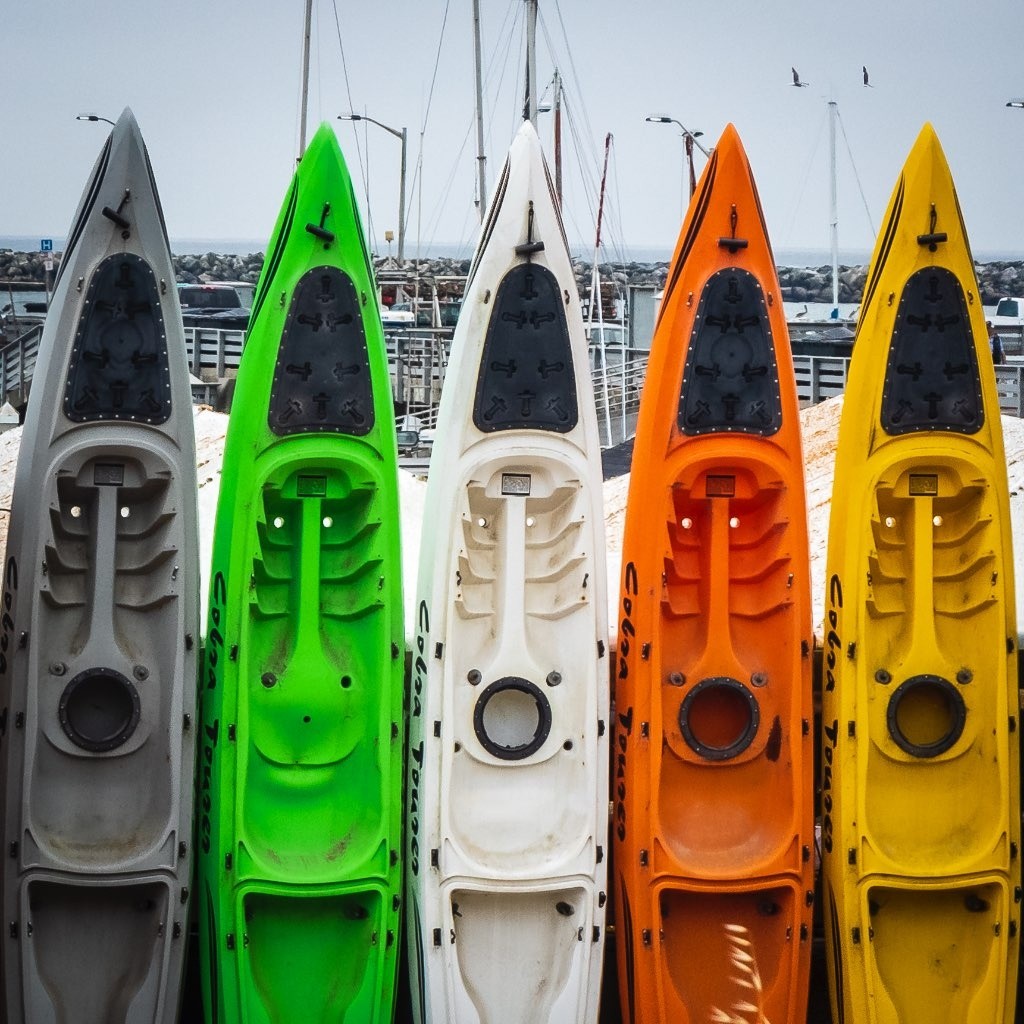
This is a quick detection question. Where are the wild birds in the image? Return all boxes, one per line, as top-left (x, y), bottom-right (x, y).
top-left (863, 65), bottom-right (873, 88)
top-left (791, 66), bottom-right (810, 88)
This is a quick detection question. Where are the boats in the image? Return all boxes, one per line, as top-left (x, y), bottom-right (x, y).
top-left (0, 103), bottom-right (201, 1023)
top-left (196, 123), bottom-right (412, 1023)
top-left (399, 115), bottom-right (613, 1024)
top-left (607, 119), bottom-right (817, 1023)
top-left (823, 121), bottom-right (1024, 1024)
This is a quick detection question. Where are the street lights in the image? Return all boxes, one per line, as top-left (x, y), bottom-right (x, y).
top-left (646, 116), bottom-right (710, 159)
top-left (338, 114), bottom-right (408, 266)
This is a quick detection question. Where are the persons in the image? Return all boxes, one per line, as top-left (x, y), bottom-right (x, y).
top-left (986, 319), bottom-right (1006, 382)
